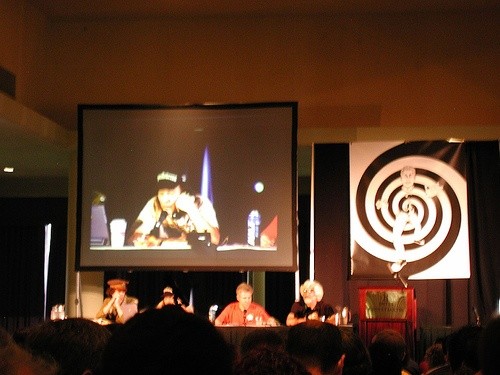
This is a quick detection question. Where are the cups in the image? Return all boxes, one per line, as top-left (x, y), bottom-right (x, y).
top-left (110, 220), bottom-right (127, 249)
top-left (255, 316), bottom-right (263, 327)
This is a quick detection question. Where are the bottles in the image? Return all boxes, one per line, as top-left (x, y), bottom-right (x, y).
top-left (247, 210), bottom-right (260, 245)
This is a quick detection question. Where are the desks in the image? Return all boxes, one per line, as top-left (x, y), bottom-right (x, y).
top-left (215, 325), bottom-right (353, 363)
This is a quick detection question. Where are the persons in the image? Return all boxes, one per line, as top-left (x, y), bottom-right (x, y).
top-left (125, 165), bottom-right (220, 247)
top-left (367, 329), bottom-right (407, 375)
top-left (231, 346), bottom-right (312, 375)
top-left (215, 283), bottom-right (278, 327)
top-left (240, 328), bottom-right (285, 354)
top-left (100, 308), bottom-right (233, 375)
top-left (157, 286), bottom-right (182, 309)
top-left (14, 328), bottom-right (30, 348)
top-left (286, 279), bottom-right (335, 326)
top-left (287, 321), bottom-right (346, 375)
top-left (95, 280), bottom-right (138, 324)
top-left (420, 318), bottom-right (500, 375)
top-left (25, 318), bottom-right (112, 375)
top-left (341, 329), bottom-right (372, 375)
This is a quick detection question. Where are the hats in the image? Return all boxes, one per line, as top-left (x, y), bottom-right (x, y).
top-left (162, 287), bottom-right (174, 297)
top-left (154, 167), bottom-right (188, 188)
top-left (108, 279), bottom-right (127, 292)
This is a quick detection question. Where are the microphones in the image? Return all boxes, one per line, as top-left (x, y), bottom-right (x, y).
top-left (243, 310), bottom-right (248, 319)
top-left (303, 307), bottom-right (311, 316)
top-left (150, 211), bottom-right (168, 237)
